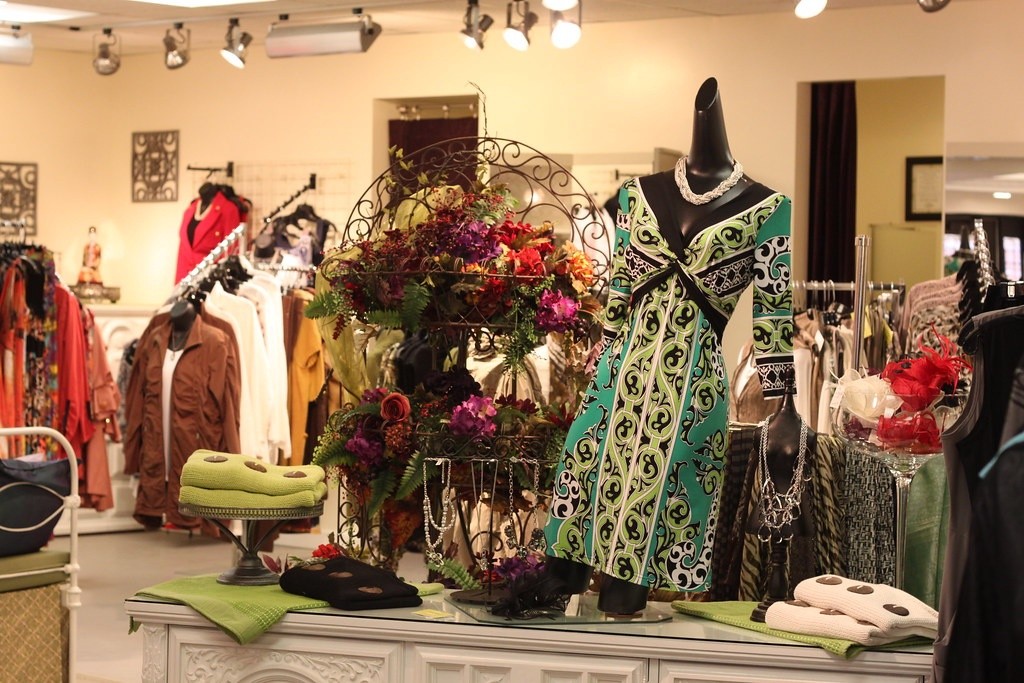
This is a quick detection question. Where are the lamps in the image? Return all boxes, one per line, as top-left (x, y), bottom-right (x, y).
top-left (263, 15), bottom-right (384, 57)
top-left (219, 19), bottom-right (253, 69)
top-left (161, 23), bottom-right (192, 68)
top-left (793, 0), bottom-right (827, 18)
top-left (918, 0), bottom-right (950, 13)
top-left (461, 0), bottom-right (584, 50)
top-left (94, 29), bottom-right (120, 73)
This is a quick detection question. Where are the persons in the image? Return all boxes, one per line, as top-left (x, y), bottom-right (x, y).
top-left (548, 77), bottom-right (793, 613)
top-left (121, 184), bottom-right (339, 528)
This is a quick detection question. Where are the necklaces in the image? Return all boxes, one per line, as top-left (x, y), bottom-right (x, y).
top-left (757, 409), bottom-right (808, 567)
top-left (675, 152), bottom-right (742, 205)
top-left (422, 455), bottom-right (541, 562)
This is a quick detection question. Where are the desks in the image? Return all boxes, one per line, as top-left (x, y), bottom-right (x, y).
top-left (0, 553), bottom-right (72, 682)
top-left (119, 603), bottom-right (933, 683)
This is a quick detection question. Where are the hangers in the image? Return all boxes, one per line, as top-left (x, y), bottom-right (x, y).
top-left (957, 226), bottom-right (999, 355)
top-left (774, 276), bottom-right (905, 334)
top-left (161, 166), bottom-right (314, 320)
top-left (1, 220), bottom-right (43, 281)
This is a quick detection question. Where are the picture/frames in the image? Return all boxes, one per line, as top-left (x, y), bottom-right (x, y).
top-left (906, 155), bottom-right (941, 221)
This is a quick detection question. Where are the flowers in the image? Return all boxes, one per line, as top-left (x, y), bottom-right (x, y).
top-left (271, 197), bottom-right (610, 610)
